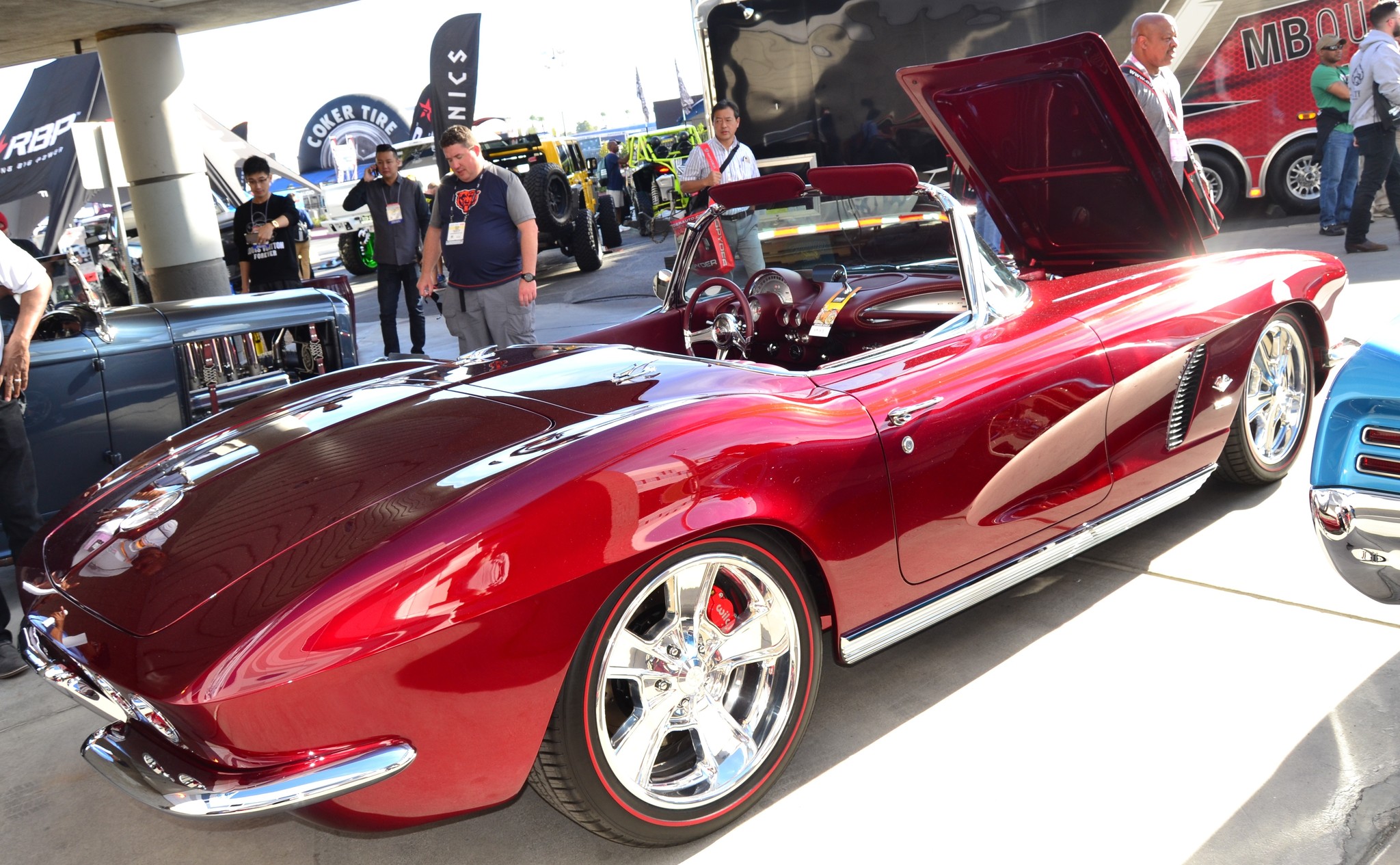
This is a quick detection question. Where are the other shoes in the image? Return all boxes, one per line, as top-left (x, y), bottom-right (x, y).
top-left (1, 638), bottom-right (31, 681)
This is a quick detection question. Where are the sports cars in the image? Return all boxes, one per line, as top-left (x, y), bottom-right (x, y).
top-left (16, 32), bottom-right (1356, 851)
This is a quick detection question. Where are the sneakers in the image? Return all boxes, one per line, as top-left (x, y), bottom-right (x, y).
top-left (1319, 224), bottom-right (1345, 236)
top-left (1337, 219), bottom-right (1349, 228)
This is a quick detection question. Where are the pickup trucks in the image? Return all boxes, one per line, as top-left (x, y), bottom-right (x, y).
top-left (322, 134), bottom-right (445, 276)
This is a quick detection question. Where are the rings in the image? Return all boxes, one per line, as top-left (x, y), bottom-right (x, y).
top-left (111, 510), bottom-right (117, 514)
top-left (14, 379), bottom-right (22, 382)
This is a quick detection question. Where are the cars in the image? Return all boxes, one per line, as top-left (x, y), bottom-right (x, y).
top-left (83, 187), bottom-right (238, 301)
top-left (1310, 334), bottom-right (1400, 606)
top-left (1, 251), bottom-right (357, 545)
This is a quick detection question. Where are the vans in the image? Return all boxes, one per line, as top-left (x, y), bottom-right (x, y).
top-left (1175, 0), bottom-right (1400, 219)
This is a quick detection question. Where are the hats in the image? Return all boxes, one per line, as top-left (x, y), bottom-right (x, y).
top-left (1316, 33), bottom-right (1347, 53)
top-left (609, 139), bottom-right (621, 145)
top-left (0, 212), bottom-right (9, 230)
top-left (286, 194), bottom-right (295, 202)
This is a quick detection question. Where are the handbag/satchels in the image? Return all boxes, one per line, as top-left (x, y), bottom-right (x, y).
top-left (1182, 152), bottom-right (1225, 239)
top-left (1373, 81), bottom-right (1400, 132)
top-left (672, 142), bottom-right (735, 277)
top-left (684, 189), bottom-right (708, 216)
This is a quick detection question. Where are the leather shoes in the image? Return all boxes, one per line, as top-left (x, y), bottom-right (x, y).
top-left (1345, 241), bottom-right (1388, 253)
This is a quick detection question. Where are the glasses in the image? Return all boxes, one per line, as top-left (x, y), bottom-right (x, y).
top-left (1321, 44), bottom-right (1343, 50)
top-left (246, 174), bottom-right (269, 185)
top-left (425, 291), bottom-right (442, 320)
top-left (6, 230), bottom-right (12, 238)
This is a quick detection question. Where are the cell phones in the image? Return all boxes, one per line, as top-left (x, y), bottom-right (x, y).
top-left (375, 167), bottom-right (379, 177)
top-left (243, 231), bottom-right (269, 245)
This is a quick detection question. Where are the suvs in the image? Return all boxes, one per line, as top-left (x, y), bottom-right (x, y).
top-left (465, 121), bottom-right (711, 274)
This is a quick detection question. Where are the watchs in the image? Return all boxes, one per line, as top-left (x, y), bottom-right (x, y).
top-left (267, 220), bottom-right (279, 231)
top-left (521, 273), bottom-right (536, 283)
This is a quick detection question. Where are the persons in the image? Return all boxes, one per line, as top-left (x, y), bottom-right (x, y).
top-left (1119, 12), bottom-right (1209, 204)
top-left (466, 346), bottom-right (557, 376)
top-left (0, 213), bottom-right (57, 337)
top-left (975, 194), bottom-right (1002, 254)
top-left (0, 229), bottom-right (52, 680)
top-left (1311, 34), bottom-right (1374, 236)
top-left (1344, 1), bottom-right (1400, 252)
top-left (297, 395), bottom-right (353, 413)
top-left (21, 486), bottom-right (177, 592)
top-left (286, 193), bottom-right (314, 281)
top-left (404, 364), bottom-right (459, 386)
top-left (424, 182), bottom-right (446, 293)
top-left (679, 101), bottom-right (766, 292)
top-left (605, 140), bottom-right (634, 233)
top-left (233, 155), bottom-right (319, 384)
top-left (27, 605), bottom-right (88, 667)
top-left (343, 144), bottom-right (431, 360)
top-left (820, 108), bottom-right (845, 168)
top-left (860, 98), bottom-right (885, 164)
top-left (416, 126), bottom-right (539, 358)
top-left (438, 255), bottom-right (446, 283)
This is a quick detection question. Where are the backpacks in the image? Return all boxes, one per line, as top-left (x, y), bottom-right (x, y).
top-left (293, 220), bottom-right (309, 243)
